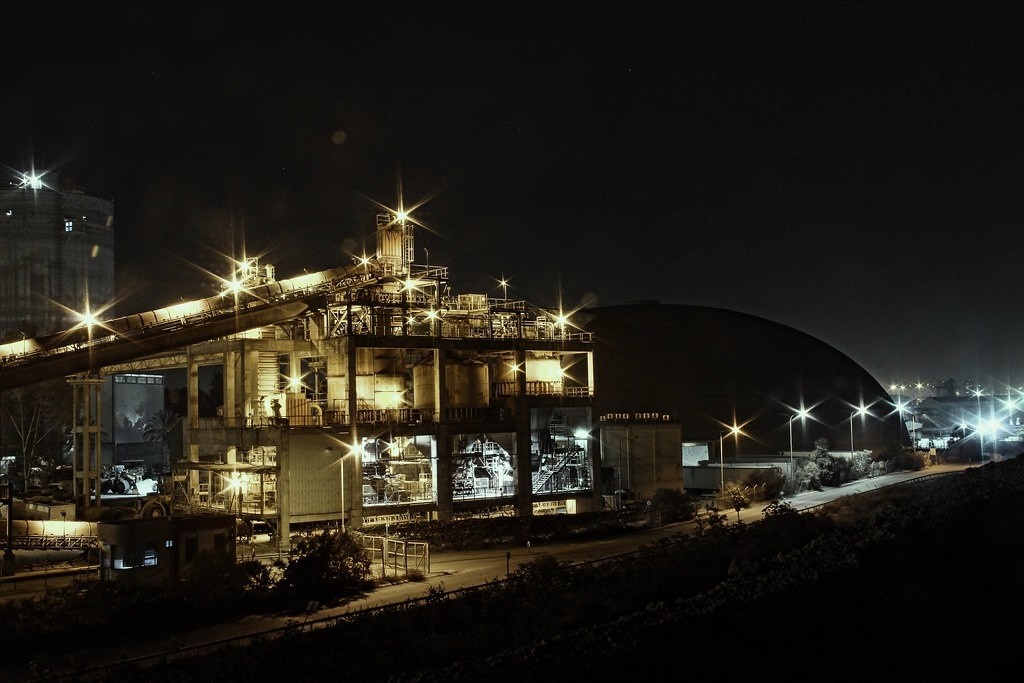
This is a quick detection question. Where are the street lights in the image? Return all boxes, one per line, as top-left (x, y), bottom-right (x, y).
top-left (720, 427), bottom-right (739, 511)
top-left (340, 445), bottom-right (362, 533)
top-left (502, 281), bottom-right (507, 309)
top-left (913, 412), bottom-right (925, 452)
top-left (620, 435), bottom-right (639, 509)
top-left (850, 408), bottom-right (866, 458)
top-left (790, 411), bottom-right (807, 497)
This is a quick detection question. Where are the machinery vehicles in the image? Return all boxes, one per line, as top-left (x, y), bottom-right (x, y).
top-left (98, 472), bottom-right (172, 522)
top-left (100, 465), bottom-right (160, 496)
